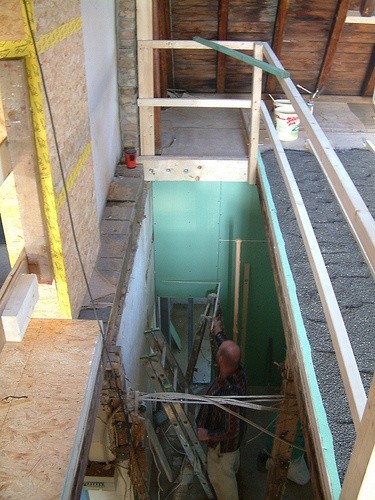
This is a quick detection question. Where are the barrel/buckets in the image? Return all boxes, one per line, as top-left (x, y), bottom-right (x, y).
top-left (273, 105), bottom-right (300, 142)
top-left (272, 99), bottom-right (293, 108)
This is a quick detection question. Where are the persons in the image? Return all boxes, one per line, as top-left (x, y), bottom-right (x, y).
top-left (193, 317), bottom-right (246, 500)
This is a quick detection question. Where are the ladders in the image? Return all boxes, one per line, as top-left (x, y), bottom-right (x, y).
top-left (141, 328), bottom-right (216, 499)
